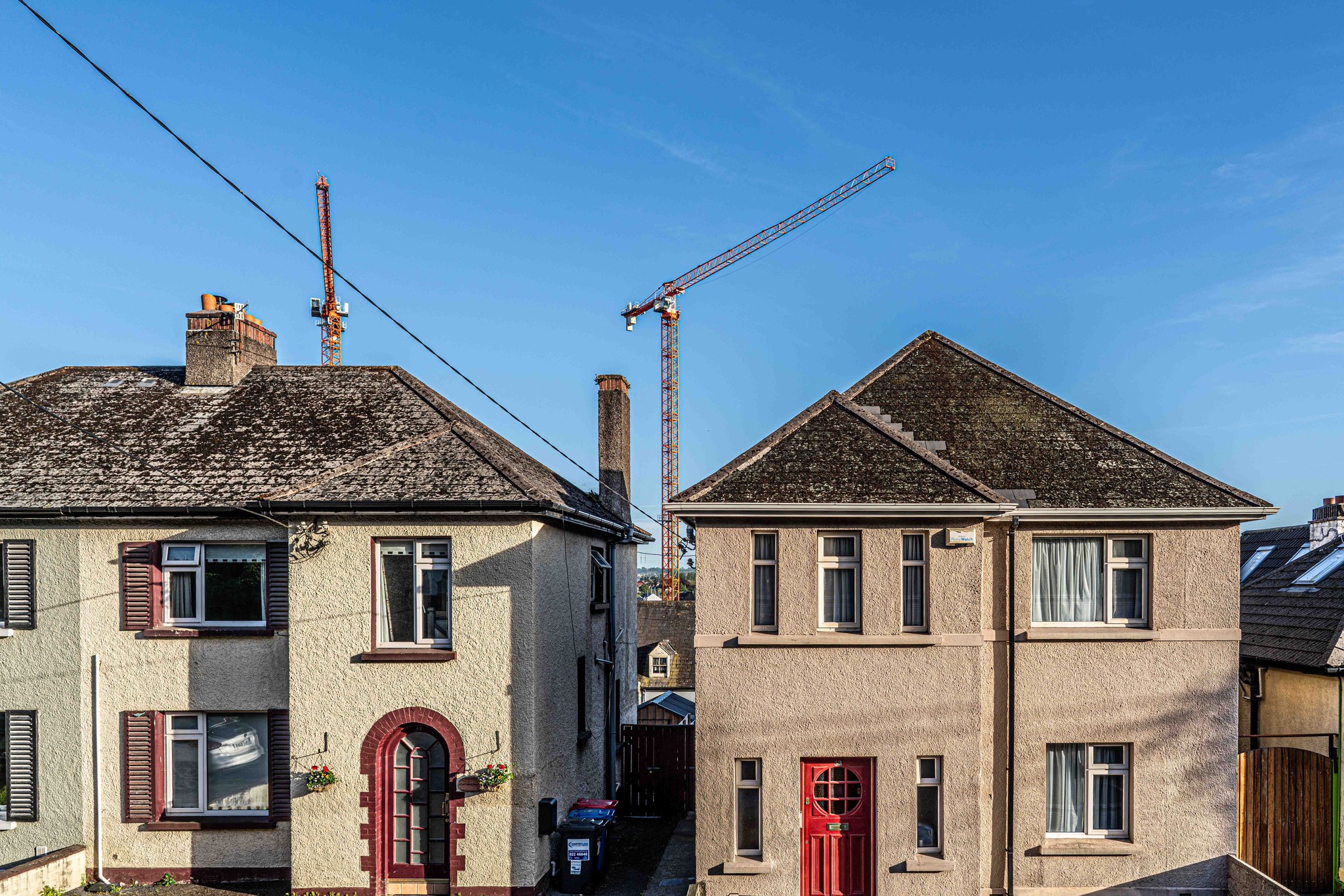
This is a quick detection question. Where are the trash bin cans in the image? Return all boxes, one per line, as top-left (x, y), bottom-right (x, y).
top-left (567, 807), bottom-right (615, 870)
top-left (556, 817), bottom-right (610, 894)
top-left (570, 798), bottom-right (619, 811)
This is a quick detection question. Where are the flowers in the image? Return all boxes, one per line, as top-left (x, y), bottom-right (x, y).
top-left (307, 765), bottom-right (340, 790)
top-left (480, 763), bottom-right (512, 783)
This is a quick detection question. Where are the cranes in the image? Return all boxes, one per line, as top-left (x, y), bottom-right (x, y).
top-left (619, 155), bottom-right (899, 602)
top-left (308, 178), bottom-right (352, 366)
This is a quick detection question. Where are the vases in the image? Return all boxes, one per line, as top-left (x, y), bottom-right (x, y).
top-left (310, 782), bottom-right (335, 793)
top-left (480, 782), bottom-right (508, 792)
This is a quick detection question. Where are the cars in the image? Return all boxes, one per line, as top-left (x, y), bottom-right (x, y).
top-left (173, 715), bottom-right (265, 779)
